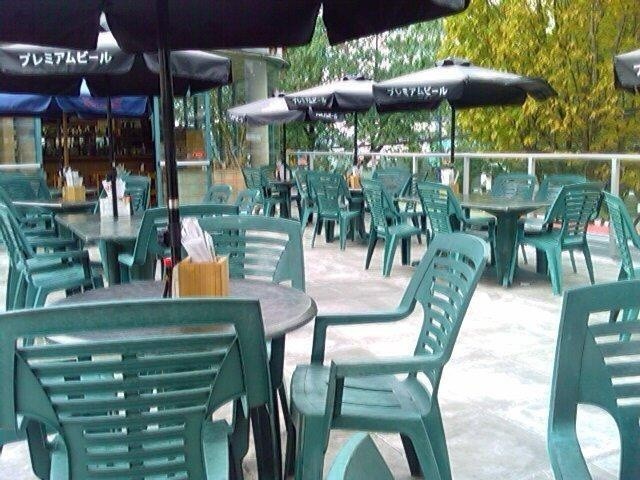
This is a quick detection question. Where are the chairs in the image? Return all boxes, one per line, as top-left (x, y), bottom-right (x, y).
top-left (602, 189), bottom-right (639, 345)
top-left (307, 172), bottom-right (361, 250)
top-left (0, 169), bottom-right (55, 310)
top-left (91, 174), bottom-right (151, 213)
top-left (510, 182), bottom-right (607, 296)
top-left (183, 215), bottom-right (303, 296)
top-left (0, 299), bottom-right (272, 479)
top-left (369, 169), bottom-right (422, 244)
top-left (285, 231), bottom-right (489, 479)
top-left (117, 205), bottom-right (241, 284)
top-left (357, 176), bottom-right (424, 277)
top-left (326, 432), bottom-right (395, 479)
top-left (491, 173), bottom-right (535, 265)
top-left (234, 188), bottom-right (260, 216)
top-left (65, 288), bottom-right (82, 298)
top-left (243, 164), bottom-right (278, 215)
top-left (534, 175), bottom-right (588, 274)
top-left (293, 170), bottom-right (323, 235)
top-left (55, 229), bottom-right (104, 288)
top-left (414, 180), bottom-right (503, 286)
top-left (545, 278), bottom-right (639, 480)
top-left (198, 184), bottom-right (233, 213)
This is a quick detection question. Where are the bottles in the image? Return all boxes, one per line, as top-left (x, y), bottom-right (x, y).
top-left (161, 258), bottom-right (173, 299)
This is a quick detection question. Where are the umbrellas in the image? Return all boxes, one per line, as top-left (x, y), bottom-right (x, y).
top-left (285, 74), bottom-right (376, 172)
top-left (612, 48), bottom-right (640, 97)
top-left (227, 91), bottom-right (338, 179)
top-left (3, 30), bottom-right (232, 223)
top-left (370, 56), bottom-right (559, 175)
top-left (0, 1), bottom-right (471, 288)
top-left (0, 79), bottom-right (152, 184)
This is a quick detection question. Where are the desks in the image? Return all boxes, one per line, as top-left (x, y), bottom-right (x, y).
top-left (461, 199), bottom-right (551, 285)
top-left (269, 179), bottom-right (296, 218)
top-left (55, 212), bottom-right (170, 289)
top-left (348, 188), bottom-right (367, 241)
top-left (42, 280), bottom-right (318, 477)
